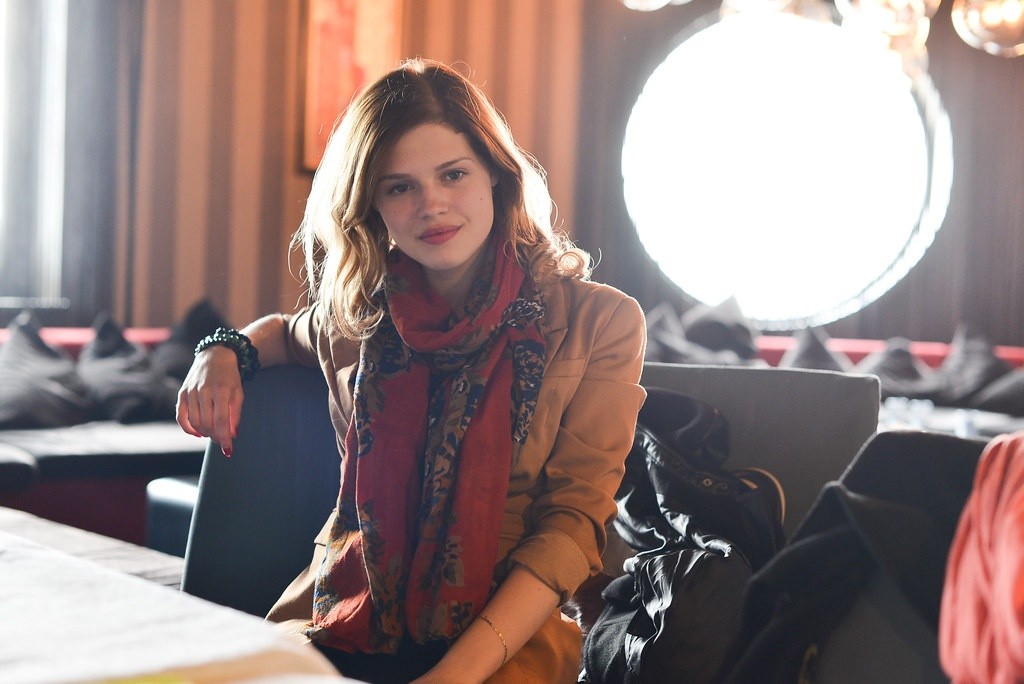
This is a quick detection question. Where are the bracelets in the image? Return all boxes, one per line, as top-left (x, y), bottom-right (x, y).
top-left (476, 614), bottom-right (508, 669)
top-left (194, 327), bottom-right (262, 380)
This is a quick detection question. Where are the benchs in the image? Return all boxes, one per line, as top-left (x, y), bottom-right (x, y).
top-left (0, 327), bottom-right (172, 361)
top-left (751, 335), bottom-right (1024, 366)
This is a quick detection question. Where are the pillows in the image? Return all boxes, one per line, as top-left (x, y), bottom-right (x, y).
top-left (638, 297), bottom-right (1024, 417)
top-left (0, 295), bottom-right (236, 430)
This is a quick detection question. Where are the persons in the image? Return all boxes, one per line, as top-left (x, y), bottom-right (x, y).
top-left (175, 55), bottom-right (649, 684)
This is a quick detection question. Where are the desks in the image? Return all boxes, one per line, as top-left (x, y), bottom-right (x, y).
top-left (0, 423), bottom-right (348, 683)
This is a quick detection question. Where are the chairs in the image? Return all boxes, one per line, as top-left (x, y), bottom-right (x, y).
top-left (713, 431), bottom-right (1024, 684)
top-left (564, 362), bottom-right (882, 582)
top-left (181, 362), bottom-right (341, 619)
